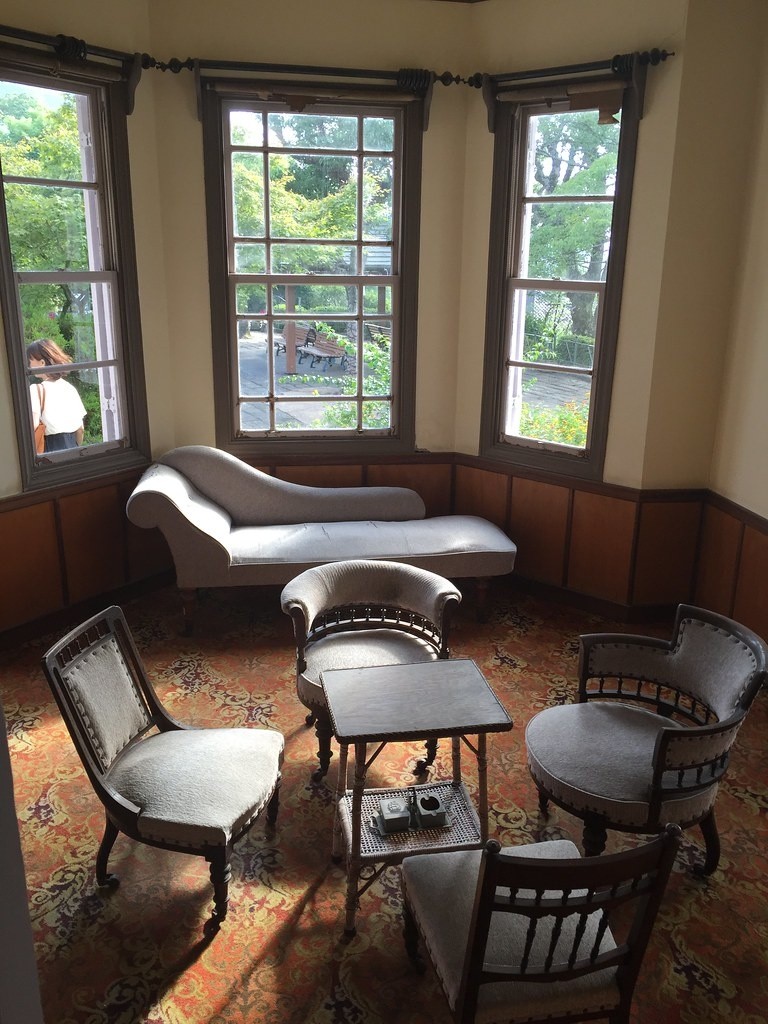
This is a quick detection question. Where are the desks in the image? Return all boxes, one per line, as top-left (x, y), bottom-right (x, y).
top-left (319, 658), bottom-right (514, 942)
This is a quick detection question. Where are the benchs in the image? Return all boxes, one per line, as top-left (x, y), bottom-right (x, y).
top-left (264, 325), bottom-right (351, 374)
top-left (365, 323), bottom-right (391, 351)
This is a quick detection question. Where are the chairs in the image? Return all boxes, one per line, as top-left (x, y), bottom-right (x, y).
top-left (281, 561), bottom-right (464, 783)
top-left (400, 821), bottom-right (685, 1024)
top-left (521, 602), bottom-right (768, 878)
top-left (40, 605), bottom-right (286, 921)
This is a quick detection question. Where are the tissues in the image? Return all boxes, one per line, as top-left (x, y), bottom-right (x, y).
top-left (379, 798), bottom-right (410, 834)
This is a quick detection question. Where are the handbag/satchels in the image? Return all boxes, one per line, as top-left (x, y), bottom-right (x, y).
top-left (35, 384), bottom-right (46, 454)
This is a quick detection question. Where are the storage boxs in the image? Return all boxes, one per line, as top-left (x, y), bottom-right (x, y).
top-left (415, 791), bottom-right (447, 828)
top-left (379, 796), bottom-right (410, 833)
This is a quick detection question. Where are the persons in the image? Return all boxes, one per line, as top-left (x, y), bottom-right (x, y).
top-left (26, 337), bottom-right (88, 454)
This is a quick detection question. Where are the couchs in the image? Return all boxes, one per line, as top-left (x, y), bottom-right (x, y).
top-left (125, 445), bottom-right (517, 623)
